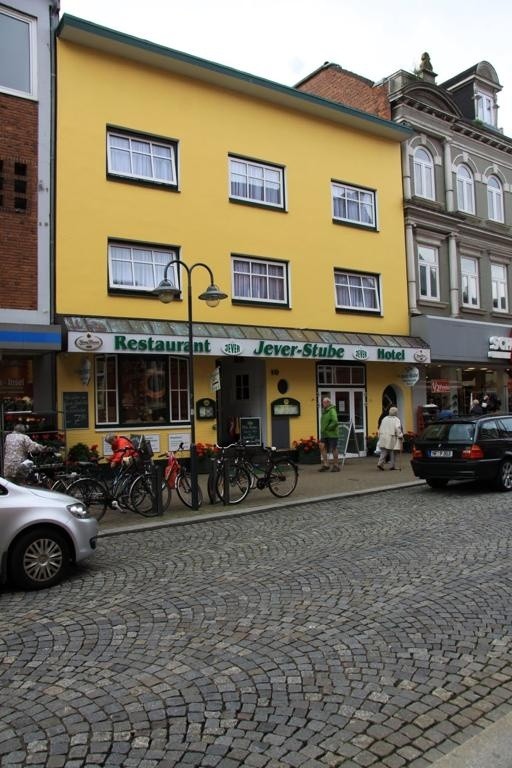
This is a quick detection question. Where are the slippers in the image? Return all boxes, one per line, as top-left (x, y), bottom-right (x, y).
top-left (318, 465), bottom-right (340, 472)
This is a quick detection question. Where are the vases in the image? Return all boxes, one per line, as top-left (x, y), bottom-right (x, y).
top-left (198, 456), bottom-right (210, 474)
top-left (299, 448), bottom-right (321, 464)
top-left (66, 462), bottom-right (98, 492)
top-left (369, 446), bottom-right (379, 457)
top-left (403, 441), bottom-right (415, 453)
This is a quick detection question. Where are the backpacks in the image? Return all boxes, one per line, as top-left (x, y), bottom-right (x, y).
top-left (120, 435), bottom-right (155, 459)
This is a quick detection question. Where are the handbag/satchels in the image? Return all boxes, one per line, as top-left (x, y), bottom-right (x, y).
top-left (478, 396), bottom-right (498, 411)
top-left (374, 441), bottom-right (381, 453)
top-left (165, 452), bottom-right (182, 488)
top-left (18, 460), bottom-right (47, 485)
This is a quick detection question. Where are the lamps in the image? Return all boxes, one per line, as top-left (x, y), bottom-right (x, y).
top-left (73, 358), bottom-right (92, 385)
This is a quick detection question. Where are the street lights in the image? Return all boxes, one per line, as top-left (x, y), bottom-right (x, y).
top-left (153, 261), bottom-right (227, 511)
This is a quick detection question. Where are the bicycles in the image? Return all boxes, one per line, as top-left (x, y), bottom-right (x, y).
top-left (8, 437), bottom-right (299, 518)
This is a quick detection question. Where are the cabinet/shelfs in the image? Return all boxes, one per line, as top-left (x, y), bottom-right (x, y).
top-left (0, 403), bottom-right (65, 488)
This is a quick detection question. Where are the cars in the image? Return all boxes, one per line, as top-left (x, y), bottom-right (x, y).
top-left (411, 412), bottom-right (510, 491)
top-left (0, 474), bottom-right (99, 589)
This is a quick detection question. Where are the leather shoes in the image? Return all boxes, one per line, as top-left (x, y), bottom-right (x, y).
top-left (377, 464), bottom-right (384, 471)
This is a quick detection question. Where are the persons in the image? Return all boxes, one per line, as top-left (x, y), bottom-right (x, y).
top-left (2, 423), bottom-right (53, 483)
top-left (376, 406), bottom-right (405, 471)
top-left (104, 432), bottom-right (143, 511)
top-left (316, 397), bottom-right (341, 472)
top-left (469, 398), bottom-right (483, 416)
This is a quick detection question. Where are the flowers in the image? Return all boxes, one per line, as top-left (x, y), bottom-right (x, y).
top-left (67, 442), bottom-right (98, 466)
top-left (293, 436), bottom-right (321, 451)
top-left (196, 443), bottom-right (220, 457)
top-left (367, 432), bottom-right (379, 448)
top-left (403, 431), bottom-right (417, 441)
